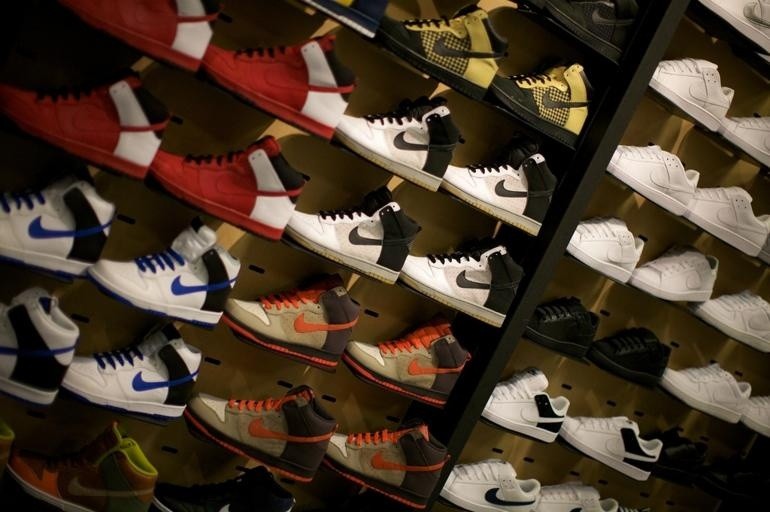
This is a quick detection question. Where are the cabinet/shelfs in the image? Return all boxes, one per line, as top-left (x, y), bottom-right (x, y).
top-left (0, 0), bottom-right (770, 512)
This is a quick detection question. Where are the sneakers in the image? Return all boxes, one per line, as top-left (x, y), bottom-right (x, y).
top-left (491, 63), bottom-right (594, 147)
top-left (61, 0), bottom-right (221, 73)
top-left (0, 164), bottom-right (116, 279)
top-left (702, 453), bottom-right (768, 508)
top-left (440, 144), bottom-right (557, 237)
top-left (87, 217), bottom-right (241, 331)
top-left (0, 420), bottom-right (16, 476)
top-left (629, 245), bottom-right (719, 301)
top-left (149, 135), bottom-right (308, 242)
top-left (686, 290), bottom-right (770, 354)
top-left (543, 0), bottom-right (638, 65)
top-left (480, 367), bottom-right (571, 443)
top-left (648, 59), bottom-right (735, 133)
top-left (152, 466), bottom-right (296, 512)
top-left (377, 4), bottom-right (509, 102)
top-left (222, 274), bottom-right (361, 373)
top-left (185, 386), bottom-right (340, 483)
top-left (559, 416), bottom-right (664, 481)
top-left (440, 460), bottom-right (541, 512)
top-left (585, 327), bottom-right (671, 388)
top-left (61, 321), bottom-right (203, 421)
top-left (535, 482), bottom-right (619, 512)
top-left (718, 117), bottom-right (770, 168)
top-left (399, 245), bottom-right (524, 328)
top-left (606, 145), bottom-right (700, 216)
top-left (683, 187), bottom-right (770, 257)
top-left (201, 34), bottom-right (359, 143)
top-left (335, 96), bottom-right (466, 194)
top-left (343, 313), bottom-right (471, 409)
top-left (658, 364), bottom-right (752, 424)
top-left (741, 396), bottom-right (770, 437)
top-left (645, 424), bottom-right (715, 487)
top-left (1, 71), bottom-right (171, 181)
top-left (525, 298), bottom-right (599, 357)
top-left (322, 423), bottom-right (447, 508)
top-left (284, 187), bottom-right (421, 285)
top-left (0, 286), bottom-right (80, 407)
top-left (566, 217), bottom-right (645, 284)
top-left (6, 421), bottom-right (158, 512)
